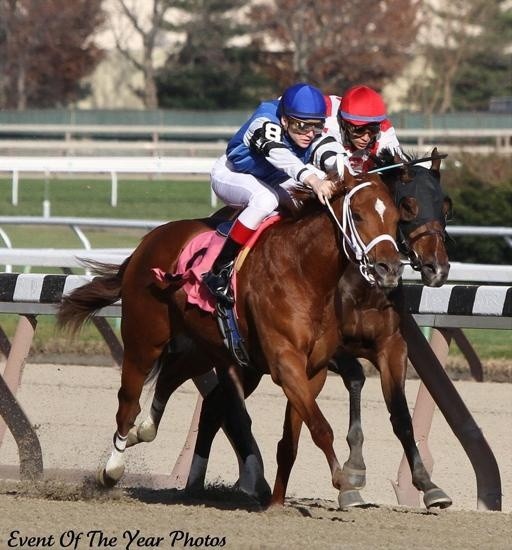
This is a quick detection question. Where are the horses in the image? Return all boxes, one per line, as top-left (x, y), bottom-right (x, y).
top-left (124, 145), bottom-right (451, 511)
top-left (55, 159), bottom-right (407, 509)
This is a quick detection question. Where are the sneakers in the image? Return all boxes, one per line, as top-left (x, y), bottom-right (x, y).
top-left (202, 260), bottom-right (235, 305)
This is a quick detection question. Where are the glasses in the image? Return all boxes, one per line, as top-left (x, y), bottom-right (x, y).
top-left (337, 115), bottom-right (379, 139)
top-left (288, 116), bottom-right (325, 136)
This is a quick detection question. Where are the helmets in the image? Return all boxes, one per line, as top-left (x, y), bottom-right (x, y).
top-left (282, 83), bottom-right (326, 120)
top-left (340, 85), bottom-right (387, 126)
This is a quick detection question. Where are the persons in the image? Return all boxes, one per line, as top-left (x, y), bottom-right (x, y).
top-left (203, 83), bottom-right (354, 305)
top-left (322, 84), bottom-right (409, 173)
